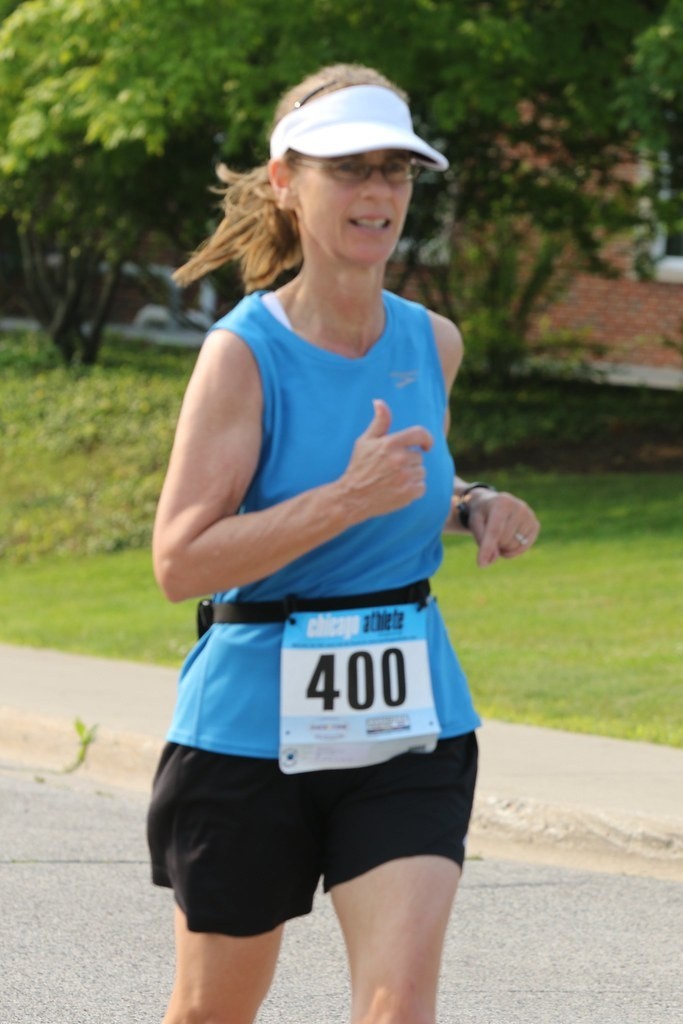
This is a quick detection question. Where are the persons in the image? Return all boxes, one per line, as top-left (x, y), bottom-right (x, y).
top-left (147, 64), bottom-right (540, 1024)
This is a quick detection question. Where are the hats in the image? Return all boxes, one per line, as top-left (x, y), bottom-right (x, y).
top-left (271, 85), bottom-right (449, 172)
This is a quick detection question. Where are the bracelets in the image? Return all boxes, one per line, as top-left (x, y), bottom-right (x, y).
top-left (457, 482), bottom-right (492, 528)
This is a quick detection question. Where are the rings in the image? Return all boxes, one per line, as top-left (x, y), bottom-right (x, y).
top-left (515, 534), bottom-right (528, 545)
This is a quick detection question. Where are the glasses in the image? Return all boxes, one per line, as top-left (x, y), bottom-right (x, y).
top-left (286, 150), bottom-right (426, 184)
